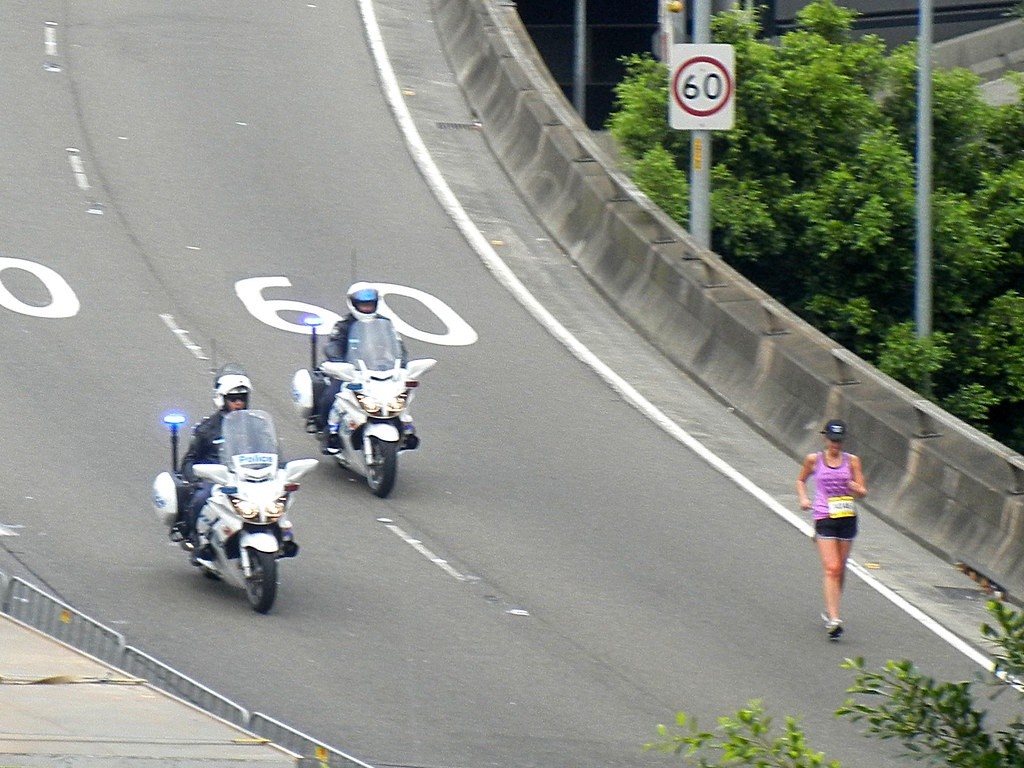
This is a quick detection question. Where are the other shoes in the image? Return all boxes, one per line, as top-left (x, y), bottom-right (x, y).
top-left (829, 624), bottom-right (842, 640)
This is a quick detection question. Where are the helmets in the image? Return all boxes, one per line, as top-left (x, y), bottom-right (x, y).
top-left (213, 374), bottom-right (254, 411)
top-left (347, 281), bottom-right (379, 321)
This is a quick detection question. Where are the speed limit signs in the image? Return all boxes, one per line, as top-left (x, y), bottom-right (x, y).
top-left (671, 56), bottom-right (732, 117)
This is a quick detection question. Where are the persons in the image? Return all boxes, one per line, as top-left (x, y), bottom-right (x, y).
top-left (183, 363), bottom-right (298, 566)
top-left (797, 419), bottom-right (867, 639)
top-left (315, 282), bottom-right (420, 455)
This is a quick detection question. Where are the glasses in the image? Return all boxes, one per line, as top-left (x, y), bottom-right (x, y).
top-left (224, 394), bottom-right (246, 402)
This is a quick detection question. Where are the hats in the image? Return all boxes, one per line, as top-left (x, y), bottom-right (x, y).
top-left (825, 420), bottom-right (846, 442)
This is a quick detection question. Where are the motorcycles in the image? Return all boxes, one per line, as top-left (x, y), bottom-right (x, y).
top-left (148, 408), bottom-right (321, 612)
top-left (288, 317), bottom-right (439, 499)
